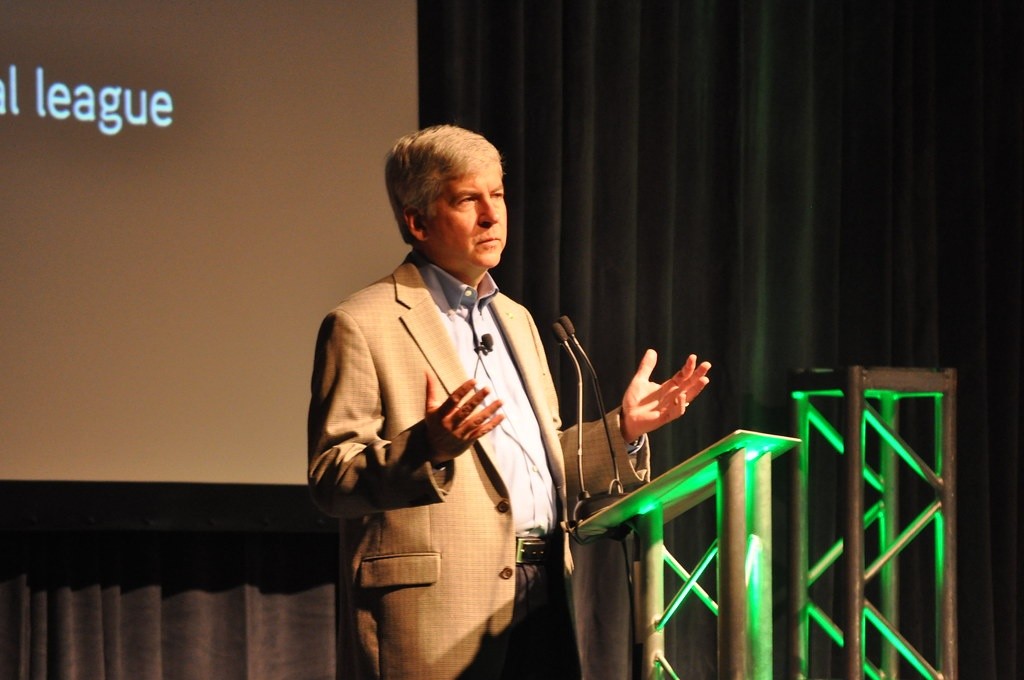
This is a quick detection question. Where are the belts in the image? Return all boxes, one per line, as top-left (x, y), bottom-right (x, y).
top-left (514, 533), bottom-right (564, 563)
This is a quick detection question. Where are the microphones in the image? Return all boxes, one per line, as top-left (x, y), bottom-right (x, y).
top-left (482, 334), bottom-right (494, 352)
top-left (552, 314), bottom-right (632, 522)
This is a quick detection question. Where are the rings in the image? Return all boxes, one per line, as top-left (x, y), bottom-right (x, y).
top-left (674, 397), bottom-right (690, 408)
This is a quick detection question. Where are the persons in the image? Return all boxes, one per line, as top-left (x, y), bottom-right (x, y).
top-left (307, 124), bottom-right (712, 680)
